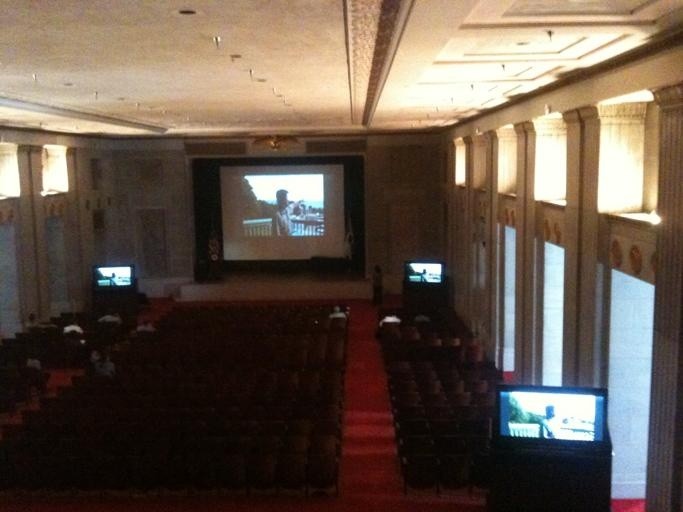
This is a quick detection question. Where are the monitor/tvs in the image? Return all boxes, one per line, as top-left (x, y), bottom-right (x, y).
top-left (495, 384), bottom-right (609, 447)
top-left (93, 264), bottom-right (135, 288)
top-left (405, 259), bottom-right (447, 284)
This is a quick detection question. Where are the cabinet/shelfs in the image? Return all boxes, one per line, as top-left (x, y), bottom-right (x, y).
top-left (402, 279), bottom-right (447, 304)
top-left (91, 278), bottom-right (138, 314)
top-left (503, 435), bottom-right (612, 509)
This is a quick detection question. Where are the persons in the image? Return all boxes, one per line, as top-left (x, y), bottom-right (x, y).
top-left (370, 265), bottom-right (383, 304)
top-left (24, 308), bottom-right (158, 343)
top-left (415, 311), bottom-right (431, 321)
top-left (539, 404), bottom-right (556, 439)
top-left (379, 311), bottom-right (400, 327)
top-left (272, 189), bottom-right (291, 237)
top-left (330, 306), bottom-right (347, 319)
top-left (110, 273), bottom-right (117, 285)
